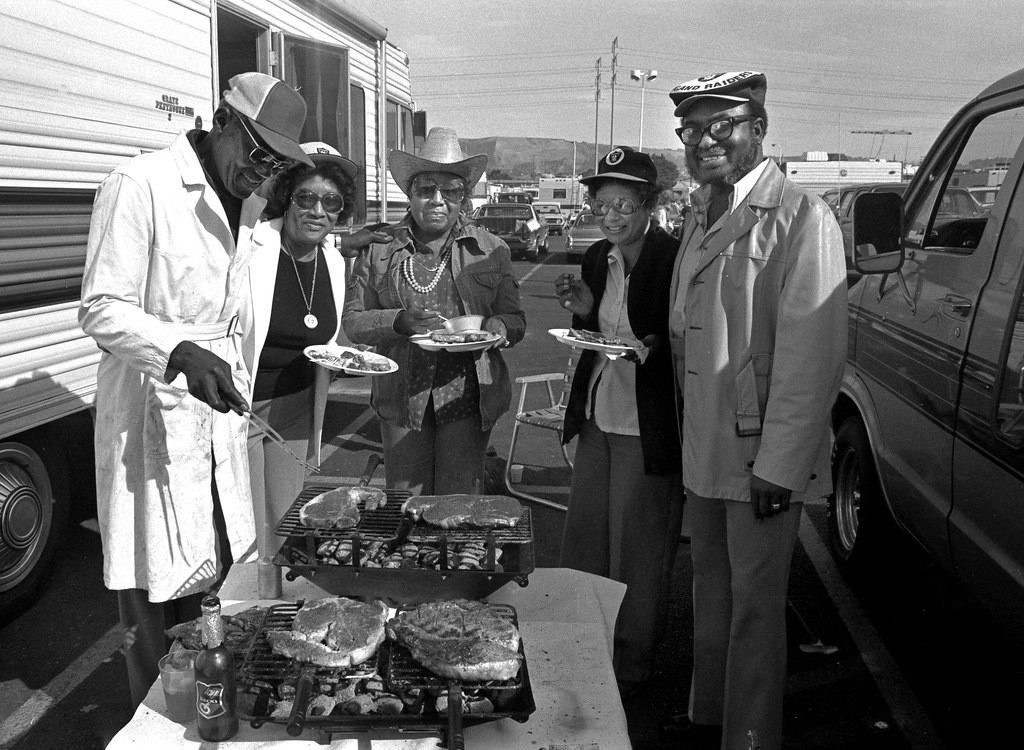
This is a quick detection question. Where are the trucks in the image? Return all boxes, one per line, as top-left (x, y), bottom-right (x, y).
top-left (0, 2), bottom-right (432, 625)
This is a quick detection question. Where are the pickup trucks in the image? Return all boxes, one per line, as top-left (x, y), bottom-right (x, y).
top-left (472, 204), bottom-right (550, 259)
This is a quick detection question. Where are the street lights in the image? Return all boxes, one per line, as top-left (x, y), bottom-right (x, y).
top-left (631, 69), bottom-right (658, 152)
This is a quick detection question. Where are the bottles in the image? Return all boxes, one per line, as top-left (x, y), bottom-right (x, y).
top-left (195, 595), bottom-right (239, 742)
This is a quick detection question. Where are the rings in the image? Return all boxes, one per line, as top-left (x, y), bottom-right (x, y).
top-left (427, 328), bottom-right (430, 332)
top-left (771, 503), bottom-right (780, 508)
top-left (503, 337), bottom-right (510, 346)
top-left (553, 291), bottom-right (560, 298)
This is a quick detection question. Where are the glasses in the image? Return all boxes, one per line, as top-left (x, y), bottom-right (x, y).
top-left (590, 197), bottom-right (647, 216)
top-left (227, 107), bottom-right (295, 172)
top-left (289, 190), bottom-right (344, 214)
top-left (410, 177), bottom-right (465, 203)
top-left (675, 113), bottom-right (757, 147)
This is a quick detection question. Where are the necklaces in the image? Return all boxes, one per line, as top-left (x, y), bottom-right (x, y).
top-left (282, 231), bottom-right (318, 328)
top-left (403, 248), bottom-right (452, 294)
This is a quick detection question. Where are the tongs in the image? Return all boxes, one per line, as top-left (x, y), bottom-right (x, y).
top-left (227, 400), bottom-right (321, 475)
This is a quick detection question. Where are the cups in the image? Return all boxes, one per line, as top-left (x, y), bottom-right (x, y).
top-left (158, 649), bottom-right (200, 723)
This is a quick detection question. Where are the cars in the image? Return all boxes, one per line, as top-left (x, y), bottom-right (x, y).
top-left (531, 202), bottom-right (565, 236)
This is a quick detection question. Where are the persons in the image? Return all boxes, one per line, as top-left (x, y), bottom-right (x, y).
top-left (669, 70), bottom-right (849, 750)
top-left (554, 146), bottom-right (685, 698)
top-left (341, 128), bottom-right (526, 495)
top-left (239, 142), bottom-right (376, 558)
top-left (77, 72), bottom-right (317, 712)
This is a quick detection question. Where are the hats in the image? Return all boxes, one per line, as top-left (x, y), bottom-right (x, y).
top-left (286, 141), bottom-right (357, 179)
top-left (223, 72), bottom-right (317, 169)
top-left (669, 70), bottom-right (766, 116)
top-left (389, 127), bottom-right (488, 197)
top-left (578, 145), bottom-right (658, 186)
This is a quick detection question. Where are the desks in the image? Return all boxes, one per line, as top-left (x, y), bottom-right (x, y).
top-left (105, 563), bottom-right (632, 750)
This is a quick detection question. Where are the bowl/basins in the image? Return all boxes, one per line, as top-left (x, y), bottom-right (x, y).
top-left (441, 314), bottom-right (485, 333)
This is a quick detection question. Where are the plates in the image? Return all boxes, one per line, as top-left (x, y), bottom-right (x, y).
top-left (303, 343), bottom-right (399, 377)
top-left (547, 328), bottom-right (643, 353)
top-left (409, 330), bottom-right (501, 351)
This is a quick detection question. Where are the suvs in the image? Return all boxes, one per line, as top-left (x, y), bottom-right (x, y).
top-left (827, 67), bottom-right (1023, 633)
top-left (824, 186), bottom-right (991, 270)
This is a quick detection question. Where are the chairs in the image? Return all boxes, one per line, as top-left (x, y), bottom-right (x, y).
top-left (504, 345), bottom-right (584, 511)
